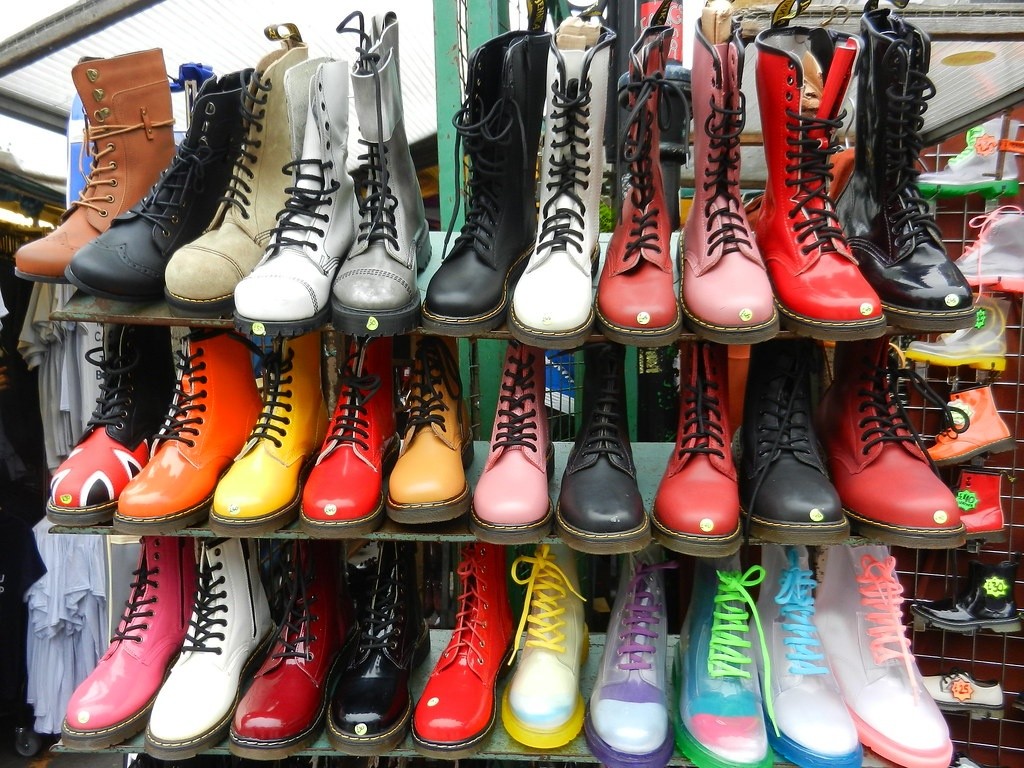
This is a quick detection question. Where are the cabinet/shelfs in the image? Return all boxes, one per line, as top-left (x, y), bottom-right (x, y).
top-left (48, 230), bottom-right (956, 768)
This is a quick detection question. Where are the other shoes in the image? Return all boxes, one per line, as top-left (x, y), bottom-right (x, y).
top-left (923, 672), bottom-right (1008, 722)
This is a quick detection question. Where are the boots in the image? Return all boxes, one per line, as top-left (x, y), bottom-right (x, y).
top-left (46, 292), bottom-right (1011, 558)
top-left (57, 538), bottom-right (1024, 768)
top-left (11, 7), bottom-right (1024, 348)
